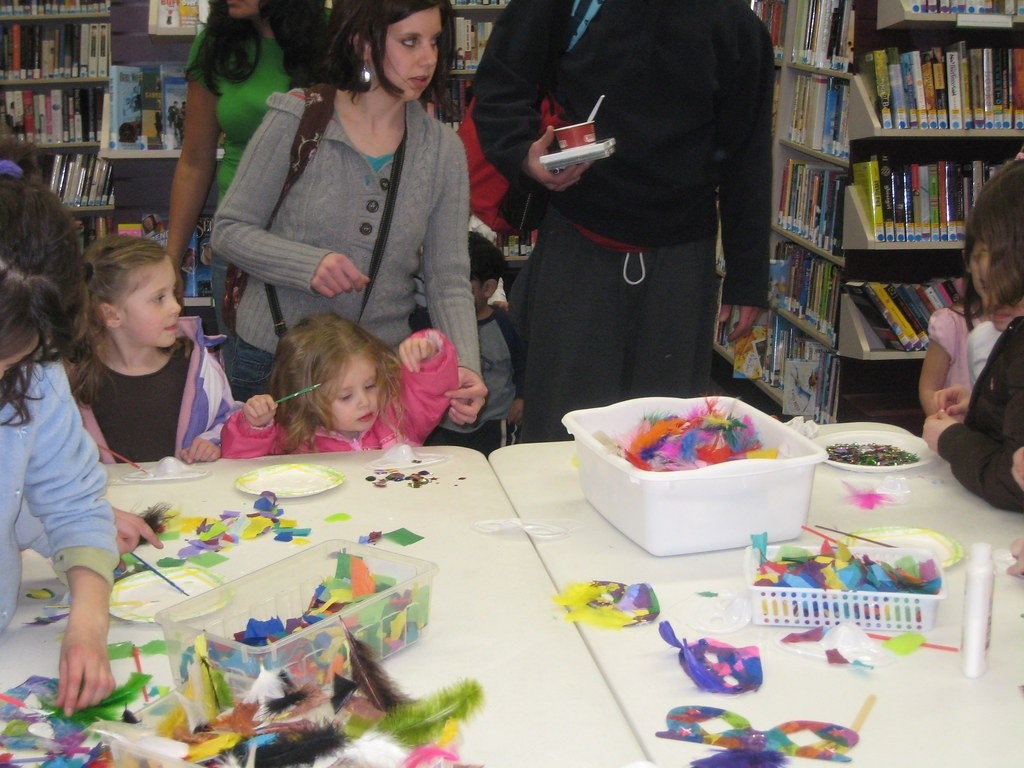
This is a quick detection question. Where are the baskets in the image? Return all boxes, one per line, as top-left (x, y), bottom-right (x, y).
top-left (744, 545), bottom-right (945, 633)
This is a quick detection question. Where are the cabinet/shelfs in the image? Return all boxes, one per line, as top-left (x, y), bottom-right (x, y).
top-left (713, 0), bottom-right (1024, 423)
top-left (0, 0), bottom-right (115, 218)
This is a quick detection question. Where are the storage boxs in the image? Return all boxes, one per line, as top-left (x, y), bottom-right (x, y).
top-left (746, 545), bottom-right (947, 631)
top-left (562, 395), bottom-right (829, 558)
top-left (156, 541), bottom-right (438, 698)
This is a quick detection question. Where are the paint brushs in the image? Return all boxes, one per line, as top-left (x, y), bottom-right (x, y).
top-left (801, 523), bottom-right (842, 545)
top-left (867, 632), bottom-right (958, 652)
top-left (274, 380), bottom-right (331, 403)
top-left (97, 442), bottom-right (157, 479)
top-left (129, 552), bottom-right (193, 598)
top-left (814, 523), bottom-right (901, 549)
top-left (0, 694), bottom-right (42, 714)
top-left (132, 645), bottom-right (149, 703)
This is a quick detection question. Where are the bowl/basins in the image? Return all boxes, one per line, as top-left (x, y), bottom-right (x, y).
top-left (554, 121), bottom-right (596, 151)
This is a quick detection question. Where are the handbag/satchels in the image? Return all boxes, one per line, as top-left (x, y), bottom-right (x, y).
top-left (221, 83), bottom-right (332, 334)
top-left (453, 81), bottom-right (570, 236)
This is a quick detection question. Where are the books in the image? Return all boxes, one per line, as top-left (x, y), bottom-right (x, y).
top-left (909, 0), bottom-right (1024, 16)
top-left (778, 155), bottom-right (851, 256)
top-left (848, 149), bottom-right (1007, 244)
top-left (772, 238), bottom-right (842, 345)
top-left (0, 0), bottom-right (112, 16)
top-left (106, 63), bottom-right (220, 306)
top-left (0, 85), bottom-right (115, 260)
top-left (714, 303), bottom-right (838, 424)
top-left (857, 39), bottom-right (1024, 130)
top-left (420, 0), bottom-right (510, 122)
top-left (843, 276), bottom-right (969, 352)
top-left (771, 63), bottom-right (852, 159)
top-left (752, 0), bottom-right (859, 75)
top-left (0, 21), bottom-right (114, 81)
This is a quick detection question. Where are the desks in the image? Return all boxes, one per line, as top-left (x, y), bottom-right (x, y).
top-left (1, 421), bottom-right (1023, 768)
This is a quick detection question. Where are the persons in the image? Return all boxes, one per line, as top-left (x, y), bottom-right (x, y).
top-left (440, 78), bottom-right (530, 456)
top-left (916, 162), bottom-right (1024, 580)
top-left (163, 1), bottom-right (328, 362)
top-left (466, 0), bottom-right (777, 446)
top-left (220, 312), bottom-right (460, 459)
top-left (66, 231), bottom-right (245, 464)
top-left (1, 135), bottom-right (165, 717)
top-left (208, 0), bottom-right (489, 429)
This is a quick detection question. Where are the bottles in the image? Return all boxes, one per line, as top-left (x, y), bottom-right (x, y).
top-left (960, 542), bottom-right (995, 678)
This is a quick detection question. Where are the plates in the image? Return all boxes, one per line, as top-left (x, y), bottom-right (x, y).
top-left (845, 527), bottom-right (963, 568)
top-left (109, 567), bottom-right (237, 623)
top-left (234, 463), bottom-right (344, 497)
top-left (813, 431), bottom-right (934, 472)
